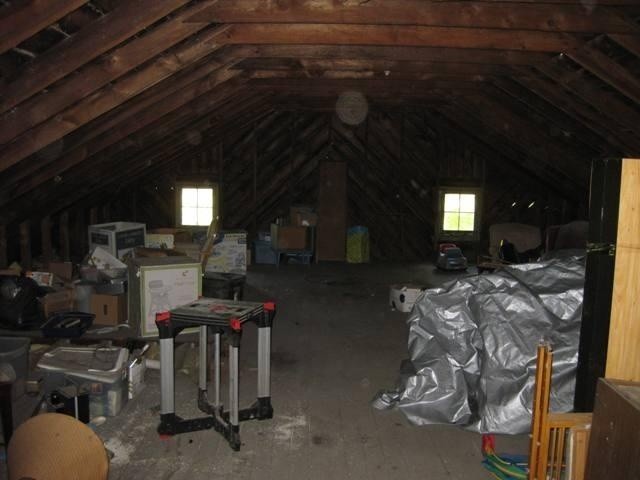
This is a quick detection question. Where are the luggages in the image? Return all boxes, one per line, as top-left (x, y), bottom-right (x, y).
top-left (389, 282), bottom-right (427, 313)
top-left (347, 226), bottom-right (370, 264)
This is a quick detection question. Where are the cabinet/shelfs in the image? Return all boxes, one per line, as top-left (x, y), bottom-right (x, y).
top-left (316, 159), bottom-right (347, 263)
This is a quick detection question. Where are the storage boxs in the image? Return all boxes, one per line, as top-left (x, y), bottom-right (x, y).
top-left (205, 233), bottom-right (251, 275)
top-left (37, 346), bottom-right (130, 417)
top-left (88, 221), bottom-right (146, 263)
top-left (0, 336), bottom-right (31, 401)
top-left (270, 223), bottom-right (307, 251)
top-left (254, 243), bottom-right (277, 265)
top-left (146, 228), bottom-right (191, 249)
top-left (90, 292), bottom-right (127, 325)
top-left (128, 256), bottom-right (203, 338)
top-left (41, 290), bottom-right (74, 318)
